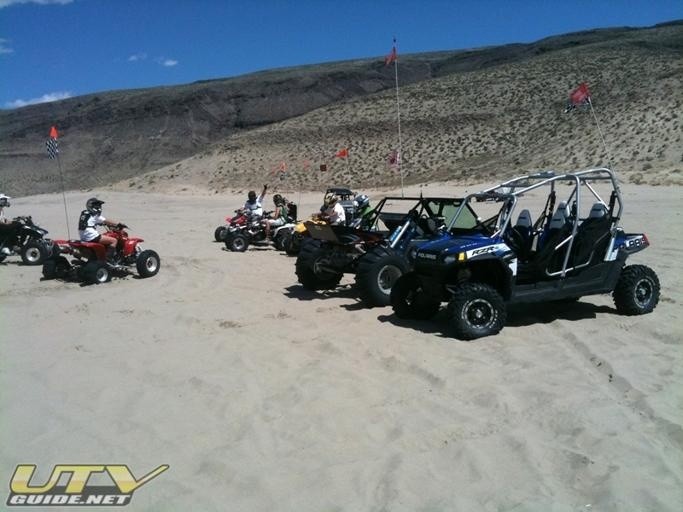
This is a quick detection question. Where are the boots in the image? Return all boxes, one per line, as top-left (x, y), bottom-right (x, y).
top-left (264, 230), bottom-right (271, 241)
top-left (104, 244), bottom-right (116, 266)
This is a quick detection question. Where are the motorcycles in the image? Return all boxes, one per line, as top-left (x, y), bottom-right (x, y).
top-left (43, 223), bottom-right (160, 284)
top-left (0, 216), bottom-right (60, 265)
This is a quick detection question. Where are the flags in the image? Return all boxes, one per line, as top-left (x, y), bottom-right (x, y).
top-left (45, 126), bottom-right (59, 159)
top-left (335, 149), bottom-right (347, 160)
top-left (268, 160), bottom-right (286, 175)
top-left (567, 83), bottom-right (591, 106)
top-left (300, 158), bottom-right (315, 169)
top-left (384, 48), bottom-right (395, 66)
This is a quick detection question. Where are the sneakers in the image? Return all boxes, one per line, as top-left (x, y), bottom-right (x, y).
top-left (1, 247), bottom-right (10, 255)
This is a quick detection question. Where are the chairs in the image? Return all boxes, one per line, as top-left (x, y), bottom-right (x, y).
top-left (511, 200), bottom-right (609, 280)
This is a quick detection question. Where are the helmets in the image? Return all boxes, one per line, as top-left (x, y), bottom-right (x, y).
top-left (0, 193), bottom-right (11, 207)
top-left (324, 192), bottom-right (338, 208)
top-left (273, 194), bottom-right (283, 205)
top-left (86, 198), bottom-right (105, 216)
top-left (248, 191), bottom-right (256, 202)
top-left (352, 194), bottom-right (370, 211)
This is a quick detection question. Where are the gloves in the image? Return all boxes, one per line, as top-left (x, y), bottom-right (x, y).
top-left (117, 223), bottom-right (128, 228)
top-left (264, 184), bottom-right (268, 190)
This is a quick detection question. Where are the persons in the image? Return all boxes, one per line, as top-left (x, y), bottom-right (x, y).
top-left (349, 194), bottom-right (378, 231)
top-left (78, 196), bottom-right (129, 272)
top-left (312, 192), bottom-right (346, 226)
top-left (263, 193), bottom-right (288, 239)
top-left (0, 193), bottom-right (15, 256)
top-left (237, 181), bottom-right (269, 219)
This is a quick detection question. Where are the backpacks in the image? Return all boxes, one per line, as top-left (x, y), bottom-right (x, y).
top-left (287, 202), bottom-right (297, 220)
